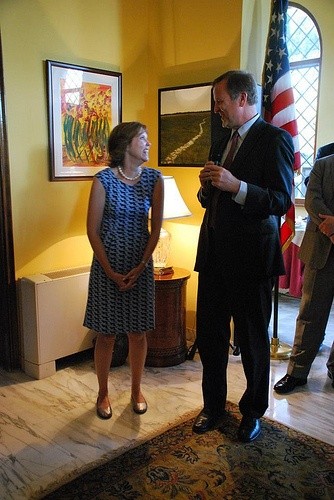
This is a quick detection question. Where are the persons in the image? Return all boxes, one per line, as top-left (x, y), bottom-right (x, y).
top-left (304, 142), bottom-right (334, 187)
top-left (191, 72), bottom-right (294, 442)
top-left (273, 151), bottom-right (334, 392)
top-left (82, 122), bottom-right (165, 420)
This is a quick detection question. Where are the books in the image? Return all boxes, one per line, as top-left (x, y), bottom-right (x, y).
top-left (153, 266), bottom-right (174, 275)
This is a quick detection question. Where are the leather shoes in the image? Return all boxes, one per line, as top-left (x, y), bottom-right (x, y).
top-left (97, 398), bottom-right (112, 419)
top-left (237, 415), bottom-right (261, 442)
top-left (274, 374), bottom-right (307, 393)
top-left (328, 370), bottom-right (334, 388)
top-left (192, 408), bottom-right (225, 432)
top-left (131, 394), bottom-right (147, 413)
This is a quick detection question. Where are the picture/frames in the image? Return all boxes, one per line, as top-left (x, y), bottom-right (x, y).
top-left (157, 82), bottom-right (213, 168)
top-left (45, 59), bottom-right (122, 182)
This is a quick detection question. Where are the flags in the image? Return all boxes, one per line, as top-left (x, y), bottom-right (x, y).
top-left (259, 0), bottom-right (302, 253)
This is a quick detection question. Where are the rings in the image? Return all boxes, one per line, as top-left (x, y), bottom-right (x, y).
top-left (212, 182), bottom-right (214, 185)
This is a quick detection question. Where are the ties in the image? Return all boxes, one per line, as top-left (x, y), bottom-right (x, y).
top-left (210, 131), bottom-right (240, 231)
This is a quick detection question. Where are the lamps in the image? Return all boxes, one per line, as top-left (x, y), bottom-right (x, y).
top-left (148, 176), bottom-right (191, 263)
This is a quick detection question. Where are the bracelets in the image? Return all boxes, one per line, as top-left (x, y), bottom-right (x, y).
top-left (142, 261), bottom-right (149, 269)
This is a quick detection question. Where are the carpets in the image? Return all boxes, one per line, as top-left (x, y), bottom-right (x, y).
top-left (30, 400), bottom-right (334, 500)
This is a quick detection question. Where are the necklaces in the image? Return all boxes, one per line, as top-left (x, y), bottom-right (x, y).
top-left (118, 164), bottom-right (142, 180)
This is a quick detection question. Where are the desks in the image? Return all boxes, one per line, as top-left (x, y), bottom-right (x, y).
top-left (278, 225), bottom-right (305, 297)
top-left (143, 267), bottom-right (190, 368)
